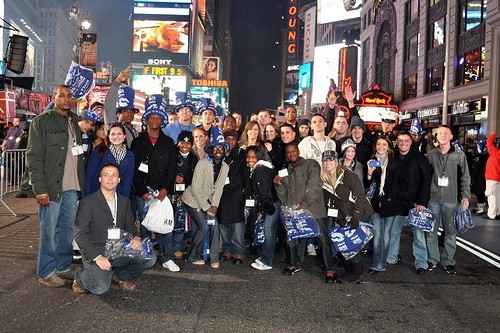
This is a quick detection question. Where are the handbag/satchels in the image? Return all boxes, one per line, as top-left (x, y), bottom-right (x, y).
top-left (140, 190), bottom-right (174, 234)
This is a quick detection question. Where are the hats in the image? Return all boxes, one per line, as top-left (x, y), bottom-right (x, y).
top-left (298, 119), bottom-right (311, 131)
top-left (350, 116), bottom-right (366, 131)
top-left (341, 139), bottom-right (356, 153)
top-left (90, 101), bottom-right (104, 111)
top-left (77, 111), bottom-right (100, 124)
top-left (204, 125), bottom-right (230, 152)
top-left (223, 129), bottom-right (238, 138)
top-left (379, 111), bottom-right (400, 123)
top-left (115, 86), bottom-right (139, 115)
top-left (198, 97), bottom-right (217, 117)
top-left (171, 92), bottom-right (197, 114)
top-left (19, 121), bottom-right (30, 130)
top-left (335, 105), bottom-right (350, 119)
top-left (142, 95), bottom-right (169, 128)
top-left (408, 117), bottom-right (426, 136)
top-left (321, 150), bottom-right (338, 160)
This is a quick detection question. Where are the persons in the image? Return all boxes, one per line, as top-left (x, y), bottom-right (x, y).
top-left (73, 163), bottom-right (156, 293)
top-left (130, 114), bottom-right (181, 272)
top-left (103, 64), bottom-right (139, 147)
top-left (318, 150), bottom-right (374, 282)
top-left (425, 125), bottom-right (473, 271)
top-left (182, 144), bottom-right (229, 266)
top-left (394, 131), bottom-right (429, 275)
top-left (274, 144), bottom-right (339, 281)
top-left (73, 85), bottom-right (440, 265)
top-left (365, 135), bottom-right (409, 277)
top-left (83, 124), bottom-right (135, 198)
top-left (0, 116), bottom-right (31, 197)
top-left (243, 147), bottom-right (285, 270)
top-left (219, 130), bottom-right (253, 263)
top-left (461, 132), bottom-right (500, 220)
top-left (25, 84), bottom-right (84, 288)
top-left (202, 58), bottom-right (217, 80)
top-left (113, 108), bottom-right (141, 149)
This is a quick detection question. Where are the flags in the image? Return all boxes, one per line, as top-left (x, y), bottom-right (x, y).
top-left (64, 59), bottom-right (95, 99)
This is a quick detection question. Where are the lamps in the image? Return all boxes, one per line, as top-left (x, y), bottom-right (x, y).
top-left (343, 0), bottom-right (362, 11)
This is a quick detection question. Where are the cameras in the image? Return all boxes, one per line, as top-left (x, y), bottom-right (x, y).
top-left (369, 161), bottom-right (381, 167)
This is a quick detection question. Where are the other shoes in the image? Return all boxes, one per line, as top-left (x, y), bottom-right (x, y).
top-left (482, 214), bottom-right (490, 219)
top-left (38, 249), bottom-right (456, 294)
top-left (14, 192), bottom-right (28, 198)
top-left (495, 214), bottom-right (500, 220)
top-left (471, 208), bottom-right (485, 216)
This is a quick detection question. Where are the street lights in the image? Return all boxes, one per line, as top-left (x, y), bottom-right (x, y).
top-left (69, 0), bottom-right (92, 65)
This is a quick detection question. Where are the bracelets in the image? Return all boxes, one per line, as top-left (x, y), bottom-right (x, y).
top-left (367, 174), bottom-right (373, 177)
